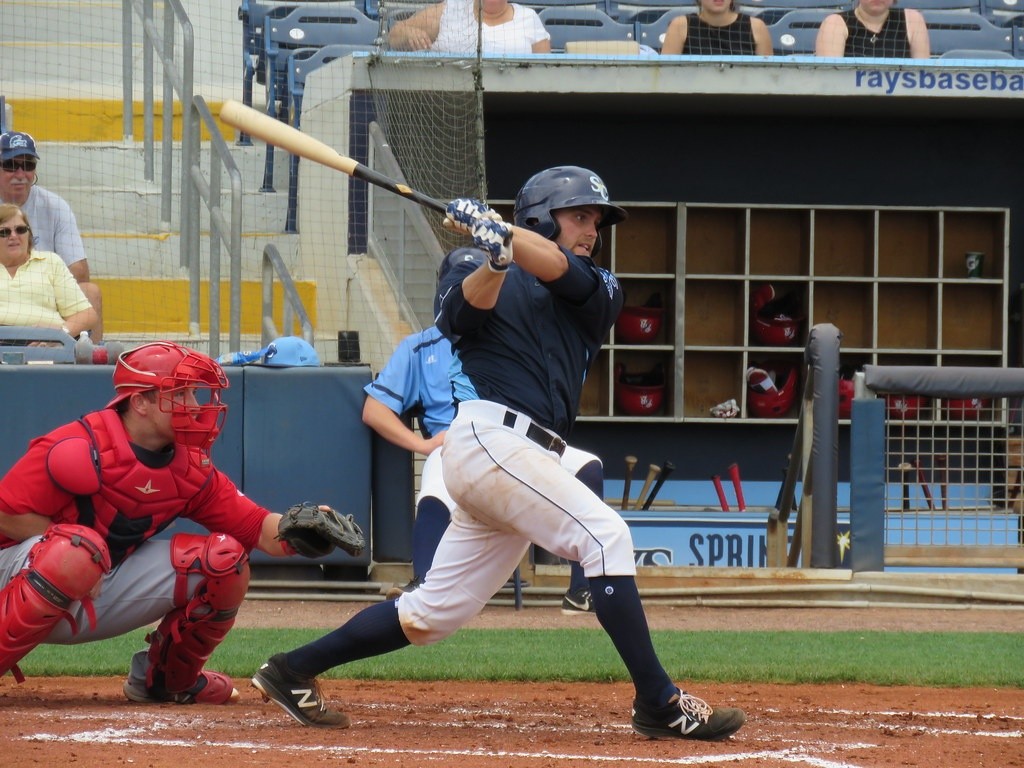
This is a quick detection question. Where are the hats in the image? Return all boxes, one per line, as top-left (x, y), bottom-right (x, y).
top-left (0, 131), bottom-right (40, 161)
top-left (250, 336), bottom-right (320, 367)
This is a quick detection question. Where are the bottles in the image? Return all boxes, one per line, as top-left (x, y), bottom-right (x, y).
top-left (93, 346), bottom-right (107, 364)
top-left (75, 330), bottom-right (93, 363)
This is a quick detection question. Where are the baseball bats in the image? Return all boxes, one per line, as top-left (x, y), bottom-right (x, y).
top-left (897, 455), bottom-right (947, 512)
top-left (218, 99), bottom-right (515, 246)
top-left (620, 455), bottom-right (747, 512)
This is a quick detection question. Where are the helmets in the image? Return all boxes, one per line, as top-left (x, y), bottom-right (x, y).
top-left (888, 395), bottom-right (927, 418)
top-left (514, 166), bottom-right (629, 240)
top-left (752, 289), bottom-right (801, 345)
top-left (942, 399), bottom-right (989, 420)
top-left (436, 247), bottom-right (487, 283)
top-left (618, 306), bottom-right (665, 344)
top-left (837, 365), bottom-right (858, 417)
top-left (615, 376), bottom-right (668, 415)
top-left (104, 341), bottom-right (186, 409)
top-left (747, 366), bottom-right (798, 418)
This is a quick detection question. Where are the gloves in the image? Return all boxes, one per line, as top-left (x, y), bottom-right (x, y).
top-left (443, 198), bottom-right (488, 235)
top-left (710, 399), bottom-right (740, 418)
top-left (472, 208), bottom-right (513, 273)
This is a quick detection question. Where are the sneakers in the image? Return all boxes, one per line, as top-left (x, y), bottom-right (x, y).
top-left (561, 585), bottom-right (596, 615)
top-left (252, 652), bottom-right (349, 730)
top-left (123, 650), bottom-right (239, 704)
top-left (632, 687), bottom-right (745, 740)
top-left (386, 575), bottom-right (424, 601)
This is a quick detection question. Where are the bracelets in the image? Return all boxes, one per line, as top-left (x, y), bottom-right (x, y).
top-left (62, 327), bottom-right (70, 334)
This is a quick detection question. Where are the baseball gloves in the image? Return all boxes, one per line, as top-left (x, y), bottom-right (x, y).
top-left (277, 503), bottom-right (366, 558)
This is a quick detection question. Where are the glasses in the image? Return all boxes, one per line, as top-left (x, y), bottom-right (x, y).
top-left (0, 226), bottom-right (30, 237)
top-left (0, 159), bottom-right (36, 171)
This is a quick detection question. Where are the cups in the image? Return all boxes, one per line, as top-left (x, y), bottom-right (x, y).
top-left (965, 251), bottom-right (986, 278)
top-left (338, 331), bottom-right (361, 362)
top-left (107, 342), bottom-right (123, 365)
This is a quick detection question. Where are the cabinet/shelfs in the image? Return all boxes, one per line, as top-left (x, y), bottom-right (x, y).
top-left (486, 199), bottom-right (1010, 427)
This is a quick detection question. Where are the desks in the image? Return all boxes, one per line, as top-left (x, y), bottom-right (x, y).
top-left (0, 364), bottom-right (373, 578)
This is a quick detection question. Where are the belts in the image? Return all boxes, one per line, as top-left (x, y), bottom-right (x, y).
top-left (503, 411), bottom-right (566, 457)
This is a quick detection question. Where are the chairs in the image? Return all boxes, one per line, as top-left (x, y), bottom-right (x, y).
top-left (236, 0), bottom-right (1023, 234)
top-left (0, 325), bottom-right (77, 364)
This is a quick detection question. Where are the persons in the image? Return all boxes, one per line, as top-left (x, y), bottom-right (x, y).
top-left (0, 131), bottom-right (103, 345)
top-left (386, 0), bottom-right (551, 54)
top-left (365, 246), bottom-right (604, 613)
top-left (249, 165), bottom-right (746, 741)
top-left (815, 0), bottom-right (930, 58)
top-left (0, 203), bottom-right (98, 348)
top-left (0, 341), bottom-right (364, 702)
top-left (661, 0), bottom-right (774, 55)
